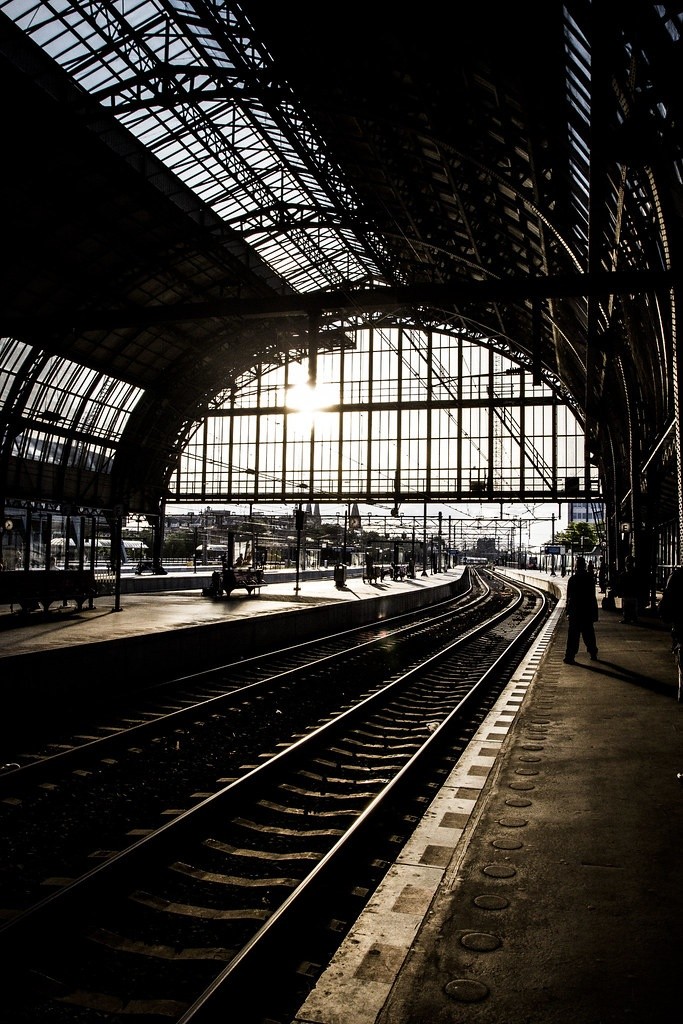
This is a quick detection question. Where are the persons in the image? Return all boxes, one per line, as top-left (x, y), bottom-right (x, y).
top-left (560, 557), bottom-right (598, 664)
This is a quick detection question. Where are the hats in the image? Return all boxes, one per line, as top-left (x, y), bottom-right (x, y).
top-left (576, 557), bottom-right (586, 567)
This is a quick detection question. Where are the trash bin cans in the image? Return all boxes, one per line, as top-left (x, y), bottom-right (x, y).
top-left (333, 563), bottom-right (347, 587)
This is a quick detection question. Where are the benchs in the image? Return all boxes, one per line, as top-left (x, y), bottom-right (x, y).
top-left (135, 562), bottom-right (162, 574)
top-left (0, 570), bottom-right (112, 611)
top-left (223, 569), bottom-right (267, 596)
top-left (202, 569), bottom-right (223, 596)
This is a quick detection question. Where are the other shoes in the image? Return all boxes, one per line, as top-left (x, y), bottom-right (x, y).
top-left (563, 655), bottom-right (574, 662)
top-left (590, 654), bottom-right (598, 660)
